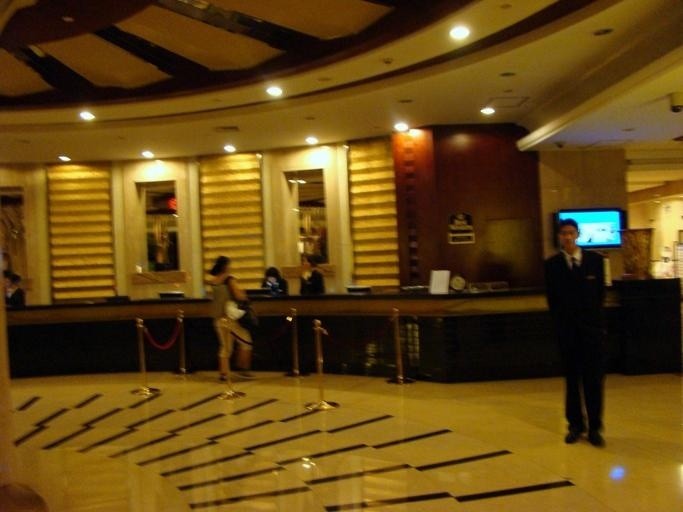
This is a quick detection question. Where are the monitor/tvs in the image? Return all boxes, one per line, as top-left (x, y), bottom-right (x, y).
top-left (245, 287), bottom-right (272, 298)
top-left (158, 291), bottom-right (185, 298)
top-left (556, 206), bottom-right (624, 251)
top-left (105, 296), bottom-right (130, 302)
top-left (347, 286), bottom-right (372, 297)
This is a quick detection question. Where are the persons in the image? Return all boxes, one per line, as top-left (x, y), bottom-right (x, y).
top-left (4, 271), bottom-right (26, 308)
top-left (301, 264), bottom-right (320, 296)
top-left (211, 256), bottom-right (255, 383)
top-left (547, 222), bottom-right (615, 450)
top-left (262, 267), bottom-right (292, 294)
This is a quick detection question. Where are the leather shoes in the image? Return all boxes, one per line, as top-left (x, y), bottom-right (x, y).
top-left (565, 429), bottom-right (601, 445)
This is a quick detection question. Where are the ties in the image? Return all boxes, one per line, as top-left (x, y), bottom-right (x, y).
top-left (570, 257), bottom-right (577, 276)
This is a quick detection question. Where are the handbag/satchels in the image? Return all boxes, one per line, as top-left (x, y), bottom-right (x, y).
top-left (238, 301), bottom-right (258, 329)
